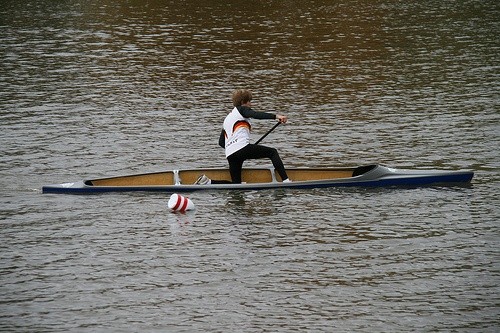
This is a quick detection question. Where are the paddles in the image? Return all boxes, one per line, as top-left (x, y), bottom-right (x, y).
top-left (253, 120), bottom-right (286, 150)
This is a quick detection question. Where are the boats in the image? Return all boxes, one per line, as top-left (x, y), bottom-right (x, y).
top-left (42, 162), bottom-right (475, 194)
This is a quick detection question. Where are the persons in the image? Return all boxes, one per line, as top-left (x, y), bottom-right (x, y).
top-left (195, 89), bottom-right (292, 184)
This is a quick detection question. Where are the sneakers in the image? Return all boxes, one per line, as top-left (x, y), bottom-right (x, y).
top-left (193, 175), bottom-right (211, 185)
